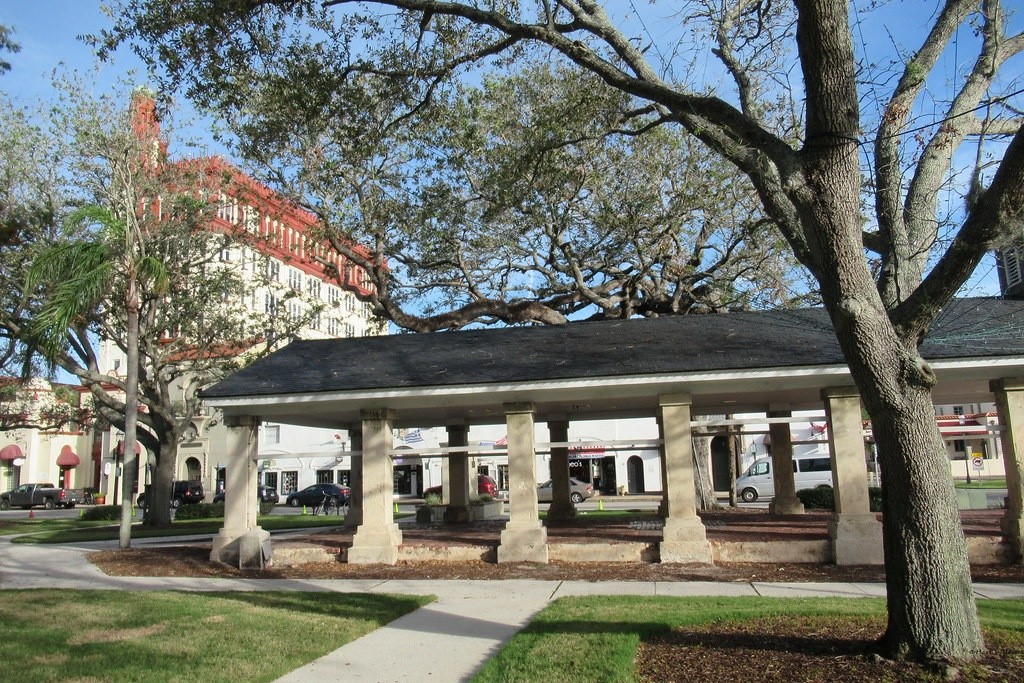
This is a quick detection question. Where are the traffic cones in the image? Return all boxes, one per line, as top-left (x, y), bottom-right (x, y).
top-left (79, 508), bottom-right (84, 517)
top-left (394, 502), bottom-right (398, 513)
top-left (597, 499), bottom-right (604, 510)
top-left (301, 505), bottom-right (308, 515)
top-left (29, 509), bottom-right (34, 518)
top-left (132, 507), bottom-right (136, 517)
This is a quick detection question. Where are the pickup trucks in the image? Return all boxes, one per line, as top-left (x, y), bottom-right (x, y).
top-left (0, 482), bottom-right (76, 511)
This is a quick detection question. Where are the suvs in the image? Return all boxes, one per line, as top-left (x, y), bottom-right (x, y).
top-left (135, 479), bottom-right (206, 509)
top-left (422, 473), bottom-right (499, 504)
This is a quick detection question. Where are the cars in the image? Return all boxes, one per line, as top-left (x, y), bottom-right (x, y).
top-left (285, 482), bottom-right (351, 507)
top-left (212, 484), bottom-right (279, 505)
top-left (537, 476), bottom-right (595, 504)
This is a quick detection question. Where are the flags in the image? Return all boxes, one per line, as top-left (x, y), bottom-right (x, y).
top-left (496, 435), bottom-right (508, 445)
top-left (404, 429), bottom-right (423, 444)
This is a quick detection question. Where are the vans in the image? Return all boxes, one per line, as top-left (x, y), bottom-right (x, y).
top-left (735, 450), bottom-right (834, 503)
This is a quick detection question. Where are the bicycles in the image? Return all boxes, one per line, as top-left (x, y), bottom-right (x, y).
top-left (312, 487), bottom-right (336, 516)
top-left (343, 483), bottom-right (352, 515)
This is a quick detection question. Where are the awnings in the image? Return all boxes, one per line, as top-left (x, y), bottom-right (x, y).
top-left (119, 439), bottom-right (141, 454)
top-left (937, 420), bottom-right (989, 436)
top-left (0, 445), bottom-right (23, 460)
top-left (56, 451), bottom-right (80, 466)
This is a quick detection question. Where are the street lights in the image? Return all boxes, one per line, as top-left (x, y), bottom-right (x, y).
top-left (958, 413), bottom-right (972, 483)
top-left (749, 441), bottom-right (758, 474)
top-left (215, 461), bottom-right (221, 495)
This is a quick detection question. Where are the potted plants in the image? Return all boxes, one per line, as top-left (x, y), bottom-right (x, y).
top-left (93, 492), bottom-right (105, 505)
